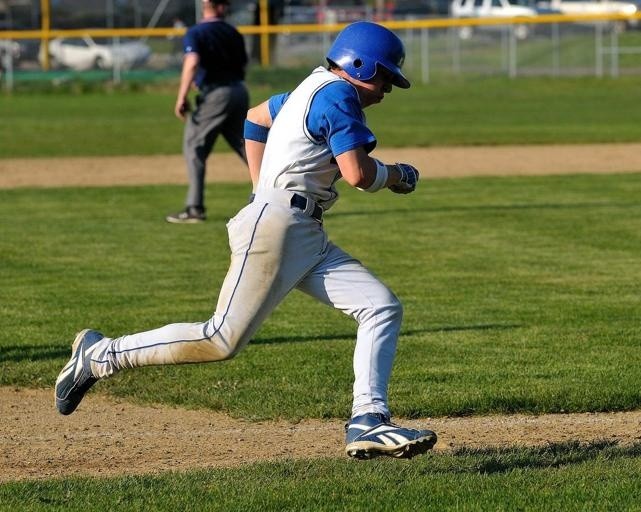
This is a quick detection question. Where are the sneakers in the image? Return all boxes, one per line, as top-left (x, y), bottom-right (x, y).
top-left (51, 327), bottom-right (106, 416)
top-left (342, 412), bottom-right (438, 461)
top-left (163, 207), bottom-right (207, 225)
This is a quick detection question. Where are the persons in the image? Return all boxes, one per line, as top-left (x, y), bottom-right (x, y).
top-left (52, 20), bottom-right (439, 462)
top-left (165, 0), bottom-right (252, 225)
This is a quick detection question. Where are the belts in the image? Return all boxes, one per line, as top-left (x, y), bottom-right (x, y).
top-left (287, 192), bottom-right (324, 222)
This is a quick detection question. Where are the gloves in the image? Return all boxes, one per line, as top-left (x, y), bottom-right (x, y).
top-left (385, 161), bottom-right (420, 196)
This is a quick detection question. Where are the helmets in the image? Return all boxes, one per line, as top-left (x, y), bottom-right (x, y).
top-left (326, 20), bottom-right (412, 90)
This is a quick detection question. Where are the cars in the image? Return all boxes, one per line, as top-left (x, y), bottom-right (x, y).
top-left (38, 36), bottom-right (151, 71)
top-left (450, 0), bottom-right (640, 41)
